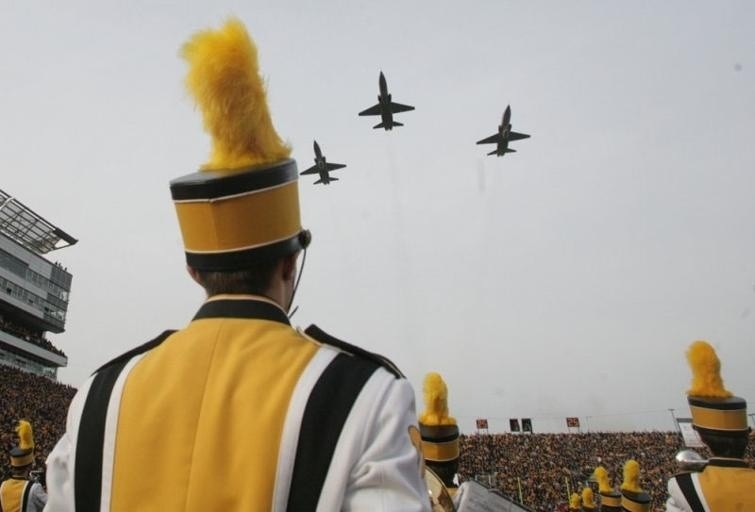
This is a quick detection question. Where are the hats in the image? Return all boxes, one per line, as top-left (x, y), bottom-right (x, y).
top-left (417, 371), bottom-right (460, 463)
top-left (687, 341), bottom-right (752, 436)
top-left (9, 419), bottom-right (35, 467)
top-left (569, 460), bottom-right (652, 511)
top-left (169, 18), bottom-right (310, 269)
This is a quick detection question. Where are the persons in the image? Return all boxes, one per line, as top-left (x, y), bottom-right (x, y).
top-left (38, 231), bottom-right (425, 511)
top-left (0, 310), bottom-right (69, 360)
top-left (2, 363), bottom-right (753, 511)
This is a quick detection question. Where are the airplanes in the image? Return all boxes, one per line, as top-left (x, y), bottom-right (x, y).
top-left (476, 105), bottom-right (531, 157)
top-left (299, 140), bottom-right (346, 185)
top-left (358, 70), bottom-right (414, 129)
